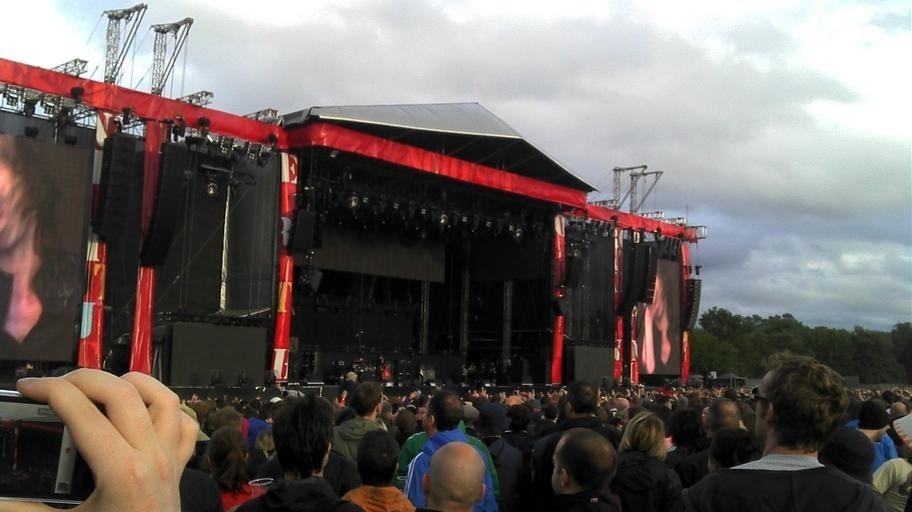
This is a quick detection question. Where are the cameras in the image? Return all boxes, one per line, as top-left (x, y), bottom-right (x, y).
top-left (0, 390), bottom-right (96, 505)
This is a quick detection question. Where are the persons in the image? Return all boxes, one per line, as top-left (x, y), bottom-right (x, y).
top-left (640, 272), bottom-right (679, 375)
top-left (1, 133), bottom-right (79, 362)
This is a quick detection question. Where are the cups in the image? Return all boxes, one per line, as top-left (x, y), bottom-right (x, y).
top-left (247, 477), bottom-right (275, 496)
top-left (397, 476), bottom-right (409, 494)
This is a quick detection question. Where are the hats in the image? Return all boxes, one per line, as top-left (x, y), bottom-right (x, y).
top-left (269, 396), bottom-right (285, 404)
top-left (820, 428), bottom-right (876, 484)
top-left (857, 398), bottom-right (889, 430)
top-left (506, 405), bottom-right (531, 433)
top-left (471, 401), bottom-right (512, 435)
top-left (893, 413), bottom-right (912, 452)
top-left (677, 395), bottom-right (689, 408)
top-left (180, 403), bottom-right (212, 442)
top-left (344, 371), bottom-right (357, 381)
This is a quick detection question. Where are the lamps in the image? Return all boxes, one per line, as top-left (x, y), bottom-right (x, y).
top-left (20, 84), bottom-right (282, 198)
top-left (308, 181), bottom-right (548, 251)
top-left (567, 210), bottom-right (689, 259)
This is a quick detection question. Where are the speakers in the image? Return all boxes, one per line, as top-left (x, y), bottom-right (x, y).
top-left (685, 280), bottom-right (701, 329)
top-left (140, 142), bottom-right (188, 266)
top-left (639, 246), bottom-right (657, 305)
top-left (293, 210), bottom-right (322, 249)
top-left (92, 133), bottom-right (137, 241)
top-left (563, 255), bottom-right (584, 287)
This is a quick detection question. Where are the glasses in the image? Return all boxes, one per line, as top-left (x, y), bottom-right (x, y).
top-left (748, 385), bottom-right (777, 410)
